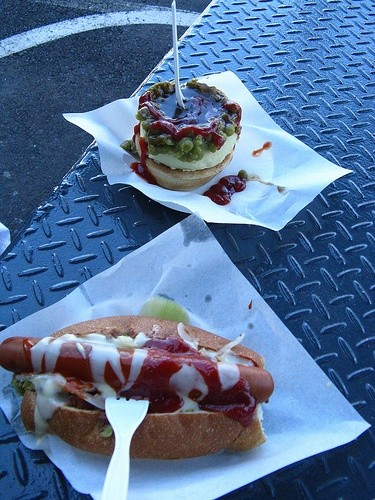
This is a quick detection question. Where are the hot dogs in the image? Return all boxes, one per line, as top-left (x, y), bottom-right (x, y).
top-left (0, 316), bottom-right (273, 460)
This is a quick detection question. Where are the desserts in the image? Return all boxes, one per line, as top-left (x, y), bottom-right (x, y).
top-left (118, 81), bottom-right (241, 190)
